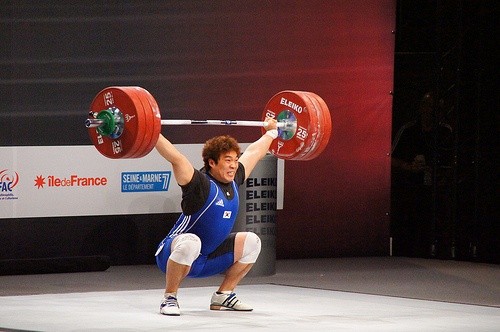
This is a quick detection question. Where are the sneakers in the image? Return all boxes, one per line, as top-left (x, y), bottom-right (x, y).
top-left (210, 291), bottom-right (254, 311)
top-left (160, 292), bottom-right (181, 316)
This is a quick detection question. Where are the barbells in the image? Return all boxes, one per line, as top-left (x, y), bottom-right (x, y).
top-left (84, 86), bottom-right (331, 161)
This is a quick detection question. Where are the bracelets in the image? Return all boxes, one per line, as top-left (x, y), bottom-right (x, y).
top-left (266, 129), bottom-right (278, 139)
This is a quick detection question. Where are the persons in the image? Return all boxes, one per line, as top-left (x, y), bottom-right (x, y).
top-left (155, 117), bottom-right (279, 316)
top-left (393, 93), bottom-right (464, 256)
